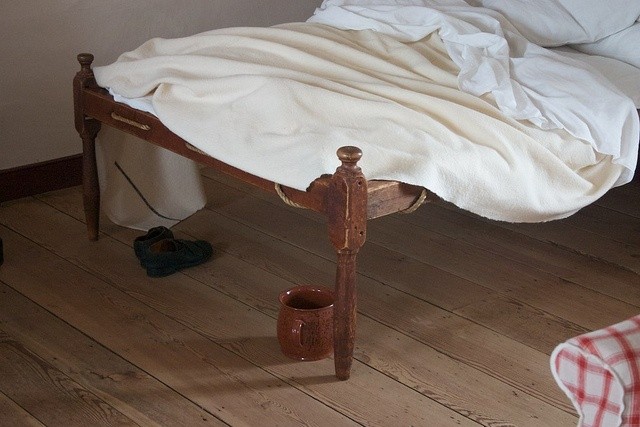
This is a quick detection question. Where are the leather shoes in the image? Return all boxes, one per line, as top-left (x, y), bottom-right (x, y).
top-left (146, 238), bottom-right (213, 278)
top-left (134, 226), bottom-right (173, 261)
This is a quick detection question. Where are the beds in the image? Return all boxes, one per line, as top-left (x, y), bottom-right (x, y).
top-left (69, 0), bottom-right (639, 383)
